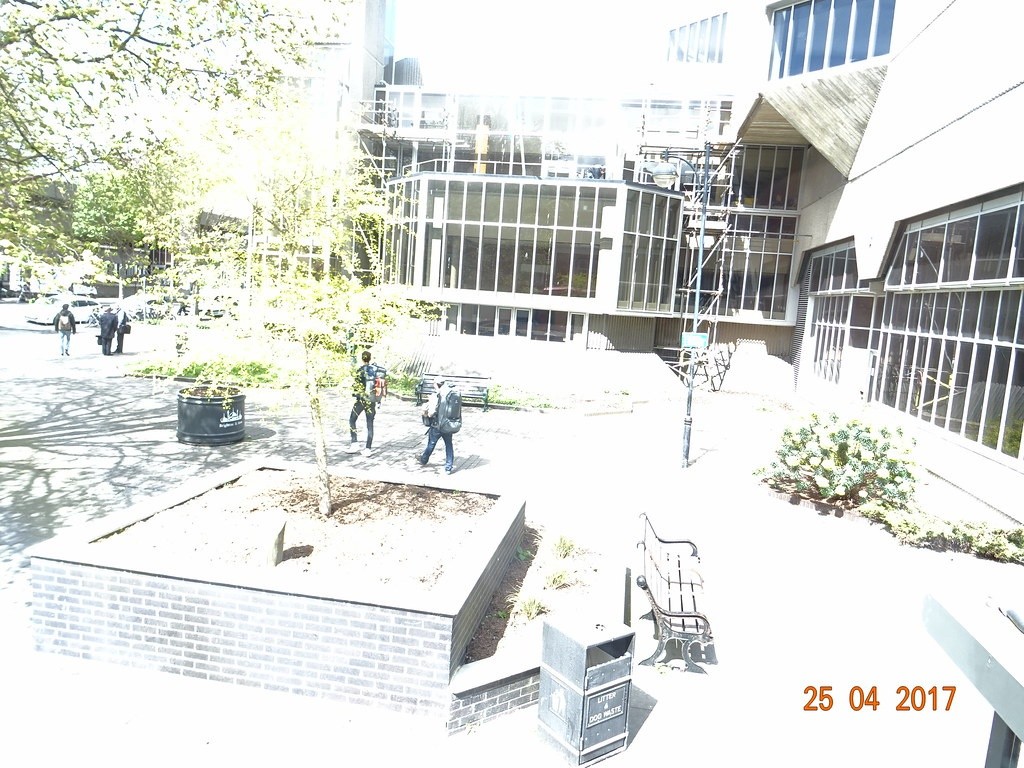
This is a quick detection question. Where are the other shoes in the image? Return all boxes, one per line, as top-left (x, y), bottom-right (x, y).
top-left (345, 442), bottom-right (359, 453)
top-left (65, 349), bottom-right (69, 356)
top-left (415, 454), bottom-right (425, 465)
top-left (445, 470), bottom-right (450, 475)
top-left (112, 350), bottom-right (122, 353)
top-left (104, 354), bottom-right (113, 356)
top-left (363, 448), bottom-right (372, 456)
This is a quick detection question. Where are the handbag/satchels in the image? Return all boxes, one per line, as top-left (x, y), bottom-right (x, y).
top-left (119, 323), bottom-right (131, 334)
top-left (95, 335), bottom-right (107, 345)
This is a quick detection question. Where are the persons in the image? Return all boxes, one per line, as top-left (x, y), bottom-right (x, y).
top-left (346, 351), bottom-right (376, 456)
top-left (414, 376), bottom-right (454, 475)
top-left (99, 305), bottom-right (127, 356)
top-left (54, 304), bottom-right (76, 356)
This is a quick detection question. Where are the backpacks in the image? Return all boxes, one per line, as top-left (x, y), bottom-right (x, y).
top-left (431, 382), bottom-right (462, 434)
top-left (58, 311), bottom-right (71, 331)
top-left (360, 362), bottom-right (386, 402)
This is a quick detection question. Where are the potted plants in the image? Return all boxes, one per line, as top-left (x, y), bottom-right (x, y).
top-left (177, 384), bottom-right (246, 445)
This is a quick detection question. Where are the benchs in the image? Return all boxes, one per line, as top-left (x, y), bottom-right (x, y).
top-left (636, 513), bottom-right (713, 674)
top-left (415, 373), bottom-right (491, 412)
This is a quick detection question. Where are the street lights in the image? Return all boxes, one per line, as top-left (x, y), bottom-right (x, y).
top-left (652, 138), bottom-right (745, 471)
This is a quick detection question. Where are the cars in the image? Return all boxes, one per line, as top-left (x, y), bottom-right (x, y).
top-left (22, 295), bottom-right (104, 326)
top-left (115, 287), bottom-right (232, 323)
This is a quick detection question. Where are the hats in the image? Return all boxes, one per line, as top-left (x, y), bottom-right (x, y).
top-left (434, 376), bottom-right (445, 385)
top-left (104, 307), bottom-right (113, 312)
